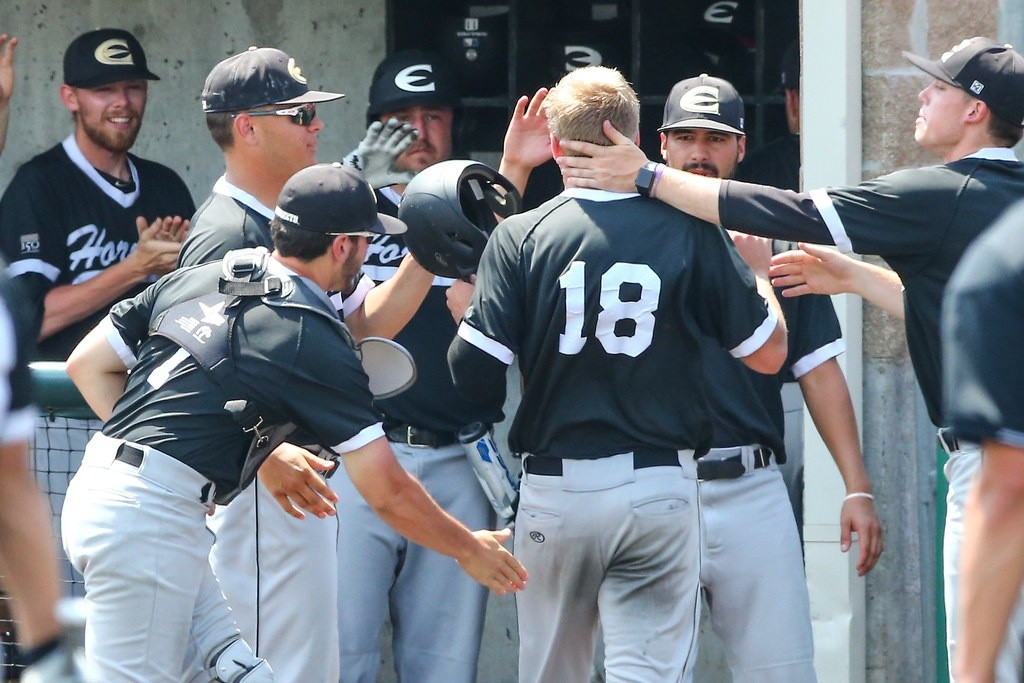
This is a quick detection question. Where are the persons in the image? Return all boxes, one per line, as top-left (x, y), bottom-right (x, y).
top-left (557, 35), bottom-right (1024, 683)
top-left (0, 267), bottom-right (85, 683)
top-left (0, 27), bottom-right (197, 596)
top-left (943, 197), bottom-right (1024, 683)
top-left (0, 33), bottom-right (19, 154)
top-left (325, 49), bottom-right (551, 683)
top-left (736, 38), bottom-right (801, 191)
top-left (658, 74), bottom-right (883, 682)
top-left (61, 162), bottom-right (529, 683)
top-left (446, 64), bottom-right (787, 683)
top-left (178, 47), bottom-right (437, 683)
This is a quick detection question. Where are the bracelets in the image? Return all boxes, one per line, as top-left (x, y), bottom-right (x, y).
top-left (649, 164), bottom-right (665, 197)
top-left (845, 493), bottom-right (874, 499)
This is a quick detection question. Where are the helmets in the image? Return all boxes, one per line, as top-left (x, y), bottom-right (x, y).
top-left (398, 160), bottom-right (522, 279)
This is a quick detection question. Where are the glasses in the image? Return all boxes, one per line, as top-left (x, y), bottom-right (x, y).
top-left (324, 231), bottom-right (376, 244)
top-left (231, 103), bottom-right (315, 125)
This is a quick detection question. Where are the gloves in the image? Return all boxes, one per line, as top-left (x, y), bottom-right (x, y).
top-left (344, 118), bottom-right (421, 189)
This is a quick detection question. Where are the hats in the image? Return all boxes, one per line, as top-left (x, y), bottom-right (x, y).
top-left (369, 50), bottom-right (461, 114)
top-left (902, 37), bottom-right (1024, 127)
top-left (549, 45), bottom-right (603, 78)
top-left (64, 29), bottom-right (160, 89)
top-left (277, 163), bottom-right (408, 235)
top-left (201, 46), bottom-right (346, 113)
top-left (657, 73), bottom-right (745, 135)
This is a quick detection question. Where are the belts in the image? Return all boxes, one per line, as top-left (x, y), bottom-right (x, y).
top-left (116, 443), bottom-right (210, 501)
top-left (526, 448), bottom-right (679, 475)
top-left (698, 447), bottom-right (770, 479)
top-left (383, 424), bottom-right (459, 448)
top-left (936, 428), bottom-right (960, 452)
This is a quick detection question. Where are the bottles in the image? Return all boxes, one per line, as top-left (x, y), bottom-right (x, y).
top-left (458, 422), bottom-right (520, 519)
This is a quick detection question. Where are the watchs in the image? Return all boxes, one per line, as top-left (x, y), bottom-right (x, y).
top-left (635, 162), bottom-right (659, 195)
